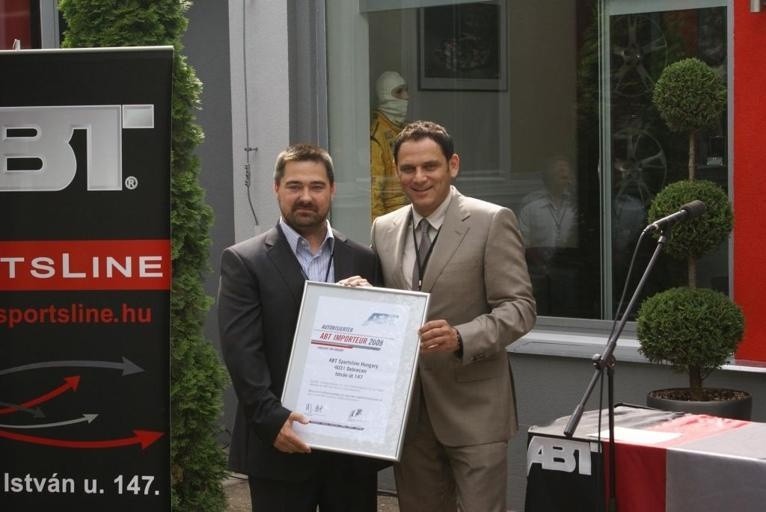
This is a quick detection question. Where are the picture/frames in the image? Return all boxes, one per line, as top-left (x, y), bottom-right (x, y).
top-left (417, 0), bottom-right (509, 93)
top-left (281, 280), bottom-right (432, 462)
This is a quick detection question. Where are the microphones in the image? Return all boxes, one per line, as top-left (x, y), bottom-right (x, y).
top-left (647, 199), bottom-right (707, 233)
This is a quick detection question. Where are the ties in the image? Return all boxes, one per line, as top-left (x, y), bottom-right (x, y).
top-left (412, 220), bottom-right (432, 291)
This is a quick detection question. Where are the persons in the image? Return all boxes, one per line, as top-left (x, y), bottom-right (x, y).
top-left (611, 157), bottom-right (654, 257)
top-left (370, 70), bottom-right (411, 226)
top-left (216, 142), bottom-right (389, 510)
top-left (521, 155), bottom-right (579, 270)
top-left (371, 120), bottom-right (537, 511)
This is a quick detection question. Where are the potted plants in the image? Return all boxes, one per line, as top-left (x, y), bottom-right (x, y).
top-left (637, 58), bottom-right (752, 420)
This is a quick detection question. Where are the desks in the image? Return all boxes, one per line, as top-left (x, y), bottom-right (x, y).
top-left (524, 406), bottom-right (766, 511)
